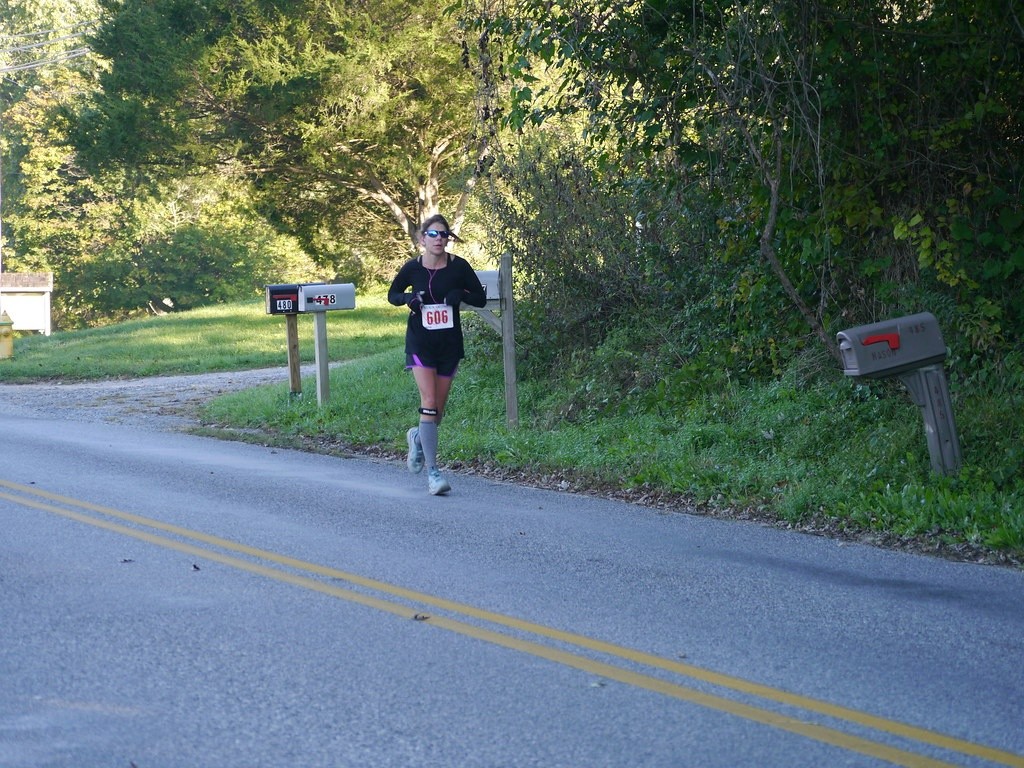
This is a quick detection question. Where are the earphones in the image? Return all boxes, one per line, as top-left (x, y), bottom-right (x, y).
top-left (422, 237), bottom-right (424, 241)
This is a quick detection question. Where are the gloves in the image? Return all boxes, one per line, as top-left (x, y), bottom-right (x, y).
top-left (409, 298), bottom-right (425, 318)
top-left (445, 288), bottom-right (462, 308)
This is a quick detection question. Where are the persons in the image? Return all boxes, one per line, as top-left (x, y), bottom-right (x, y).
top-left (387, 213), bottom-right (487, 495)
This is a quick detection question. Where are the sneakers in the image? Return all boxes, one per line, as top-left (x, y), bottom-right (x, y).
top-left (406, 426), bottom-right (425, 474)
top-left (428, 472), bottom-right (451, 495)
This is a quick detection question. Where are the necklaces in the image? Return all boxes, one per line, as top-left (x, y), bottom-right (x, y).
top-left (427, 268), bottom-right (438, 304)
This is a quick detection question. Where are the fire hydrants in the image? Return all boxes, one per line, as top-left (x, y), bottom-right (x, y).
top-left (0, 310), bottom-right (15, 361)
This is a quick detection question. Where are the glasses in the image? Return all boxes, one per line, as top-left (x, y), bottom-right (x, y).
top-left (422, 230), bottom-right (449, 238)
